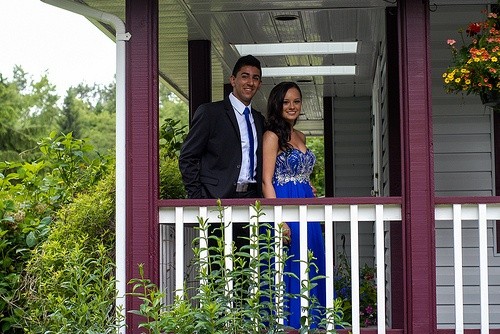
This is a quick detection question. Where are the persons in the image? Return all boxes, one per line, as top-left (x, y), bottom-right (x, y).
top-left (245, 81), bottom-right (344, 330)
top-left (179, 54), bottom-right (268, 319)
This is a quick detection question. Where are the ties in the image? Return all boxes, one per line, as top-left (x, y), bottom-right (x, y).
top-left (243, 107), bottom-right (254, 181)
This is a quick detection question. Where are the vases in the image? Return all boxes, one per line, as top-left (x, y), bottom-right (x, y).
top-left (480, 91), bottom-right (500, 106)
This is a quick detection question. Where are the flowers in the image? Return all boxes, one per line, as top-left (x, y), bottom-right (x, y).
top-left (442, 8), bottom-right (500, 96)
top-left (364, 272), bottom-right (374, 327)
